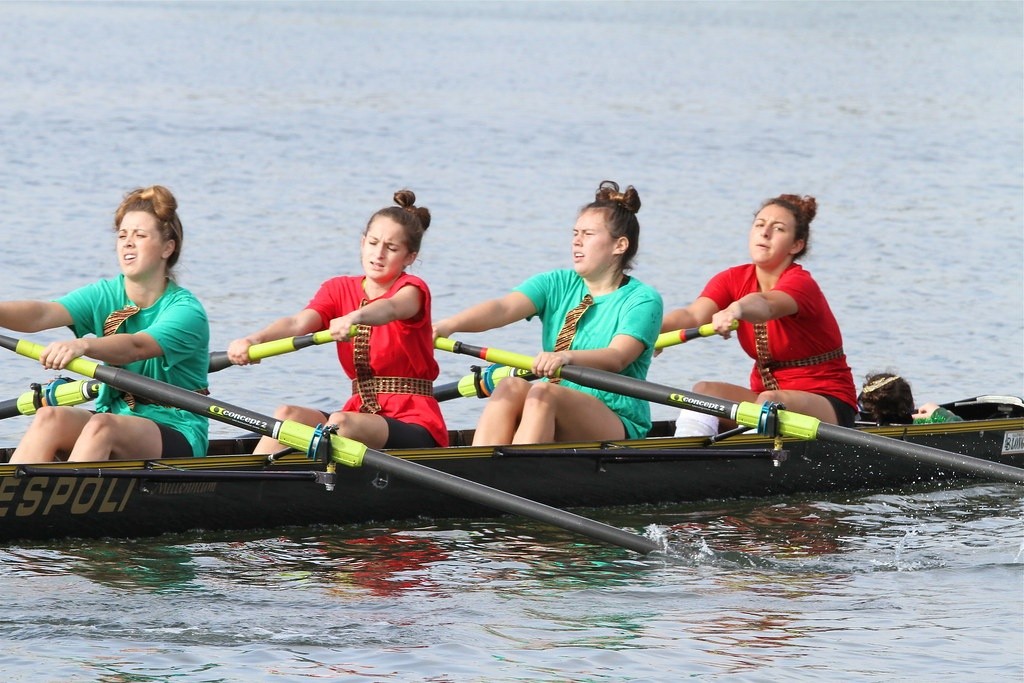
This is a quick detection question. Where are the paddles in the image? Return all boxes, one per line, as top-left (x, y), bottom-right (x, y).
top-left (0, 324), bottom-right (359, 421)
top-left (433, 335), bottom-right (1024, 486)
top-left (431, 318), bottom-right (740, 403)
top-left (0, 334), bottom-right (669, 559)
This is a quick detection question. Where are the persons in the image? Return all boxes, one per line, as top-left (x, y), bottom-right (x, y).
top-left (653, 193), bottom-right (858, 437)
top-left (228, 190), bottom-right (449, 455)
top-left (0, 186), bottom-right (211, 463)
top-left (432, 180), bottom-right (664, 447)
top-left (857, 373), bottom-right (964, 424)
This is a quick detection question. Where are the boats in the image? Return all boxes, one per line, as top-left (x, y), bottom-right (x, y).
top-left (0, 395), bottom-right (1024, 533)
